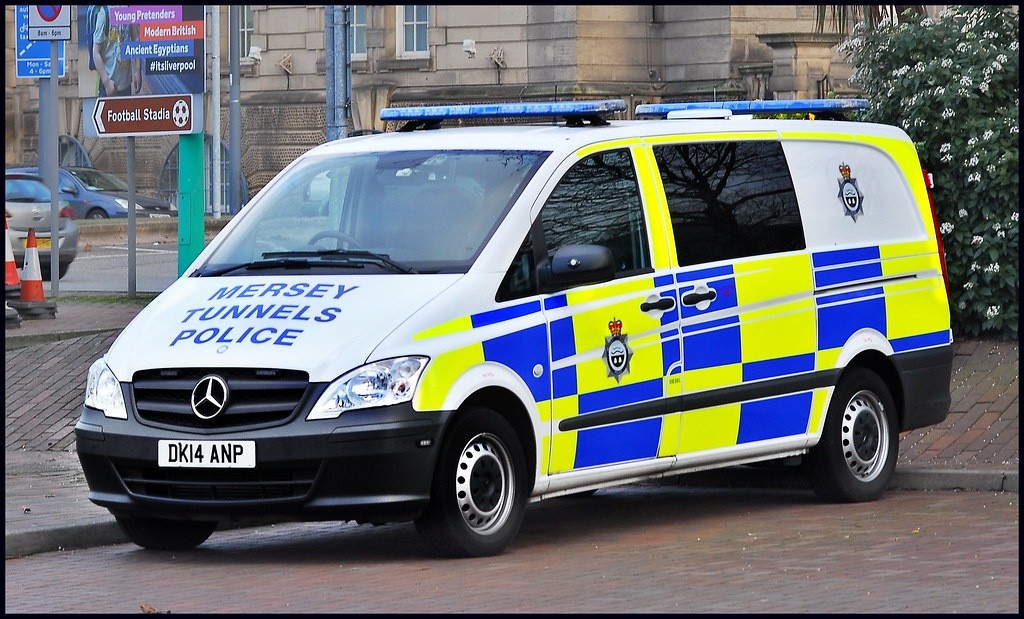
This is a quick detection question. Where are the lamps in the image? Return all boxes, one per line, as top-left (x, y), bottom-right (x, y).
top-left (463, 39), bottom-right (475, 58)
top-left (247, 46), bottom-right (260, 60)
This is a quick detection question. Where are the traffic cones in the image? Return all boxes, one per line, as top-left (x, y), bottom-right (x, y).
top-left (2, 210), bottom-right (60, 330)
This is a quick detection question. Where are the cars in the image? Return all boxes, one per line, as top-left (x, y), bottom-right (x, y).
top-left (4, 173), bottom-right (78, 281)
top-left (5, 166), bottom-right (148, 219)
top-left (66, 165), bottom-right (179, 217)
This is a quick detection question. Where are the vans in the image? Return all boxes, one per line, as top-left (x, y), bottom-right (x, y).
top-left (73, 93), bottom-right (955, 559)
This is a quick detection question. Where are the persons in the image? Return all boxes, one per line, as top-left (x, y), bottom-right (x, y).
top-left (93, 5), bottom-right (142, 98)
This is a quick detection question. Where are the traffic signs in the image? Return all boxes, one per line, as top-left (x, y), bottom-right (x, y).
top-left (15, 5), bottom-right (65, 78)
top-left (92, 94), bottom-right (192, 137)
